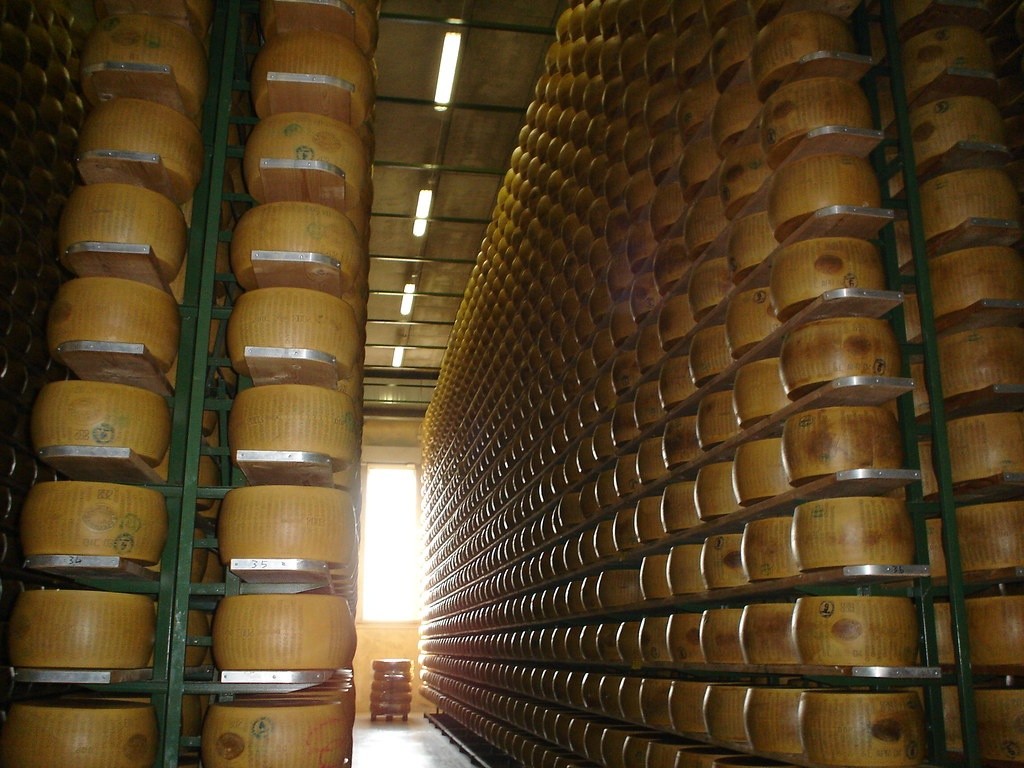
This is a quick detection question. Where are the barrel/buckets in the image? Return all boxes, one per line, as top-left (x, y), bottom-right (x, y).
top-left (0, 0), bottom-right (1024, 768)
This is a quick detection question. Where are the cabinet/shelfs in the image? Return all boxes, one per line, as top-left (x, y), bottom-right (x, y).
top-left (420, 0), bottom-right (1024, 768)
top-left (10, 0), bottom-right (354, 767)
